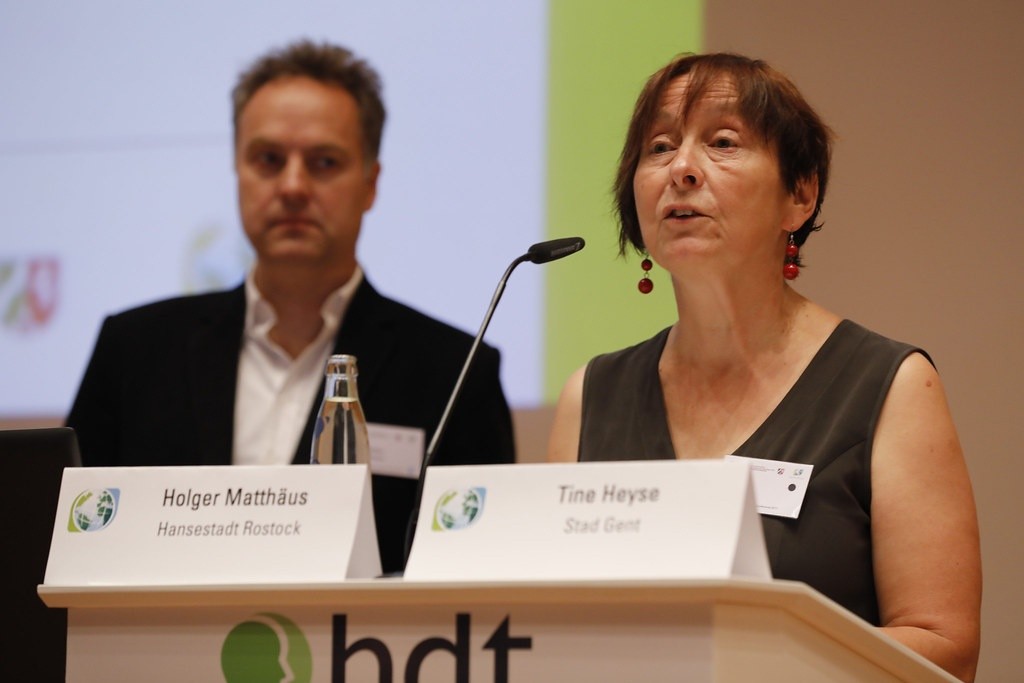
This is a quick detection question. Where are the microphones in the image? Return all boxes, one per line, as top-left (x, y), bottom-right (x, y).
top-left (403, 235), bottom-right (586, 566)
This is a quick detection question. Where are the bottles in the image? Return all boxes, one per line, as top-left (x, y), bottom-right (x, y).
top-left (309, 355), bottom-right (372, 471)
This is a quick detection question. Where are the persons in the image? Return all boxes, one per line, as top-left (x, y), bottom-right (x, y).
top-left (544, 50), bottom-right (984, 681)
top-left (59, 40), bottom-right (511, 577)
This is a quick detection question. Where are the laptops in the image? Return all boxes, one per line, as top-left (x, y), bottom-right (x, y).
top-left (0, 427), bottom-right (84, 683)
